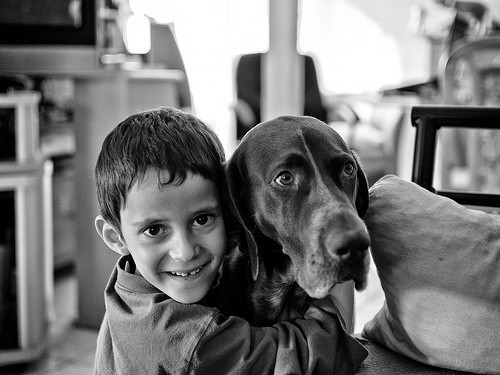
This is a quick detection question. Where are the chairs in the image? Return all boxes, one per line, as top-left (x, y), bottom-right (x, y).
top-left (357, 106), bottom-right (500, 375)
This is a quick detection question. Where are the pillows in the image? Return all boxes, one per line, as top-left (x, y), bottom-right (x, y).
top-left (359, 173), bottom-right (500, 375)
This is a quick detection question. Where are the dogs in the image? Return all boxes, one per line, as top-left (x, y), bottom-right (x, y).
top-left (207, 114), bottom-right (373, 329)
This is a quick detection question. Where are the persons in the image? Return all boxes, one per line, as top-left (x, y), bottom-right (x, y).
top-left (94, 106), bottom-right (369, 375)
